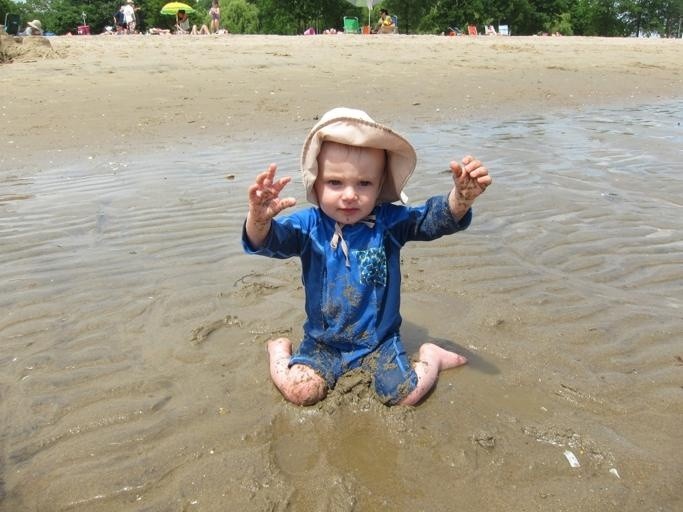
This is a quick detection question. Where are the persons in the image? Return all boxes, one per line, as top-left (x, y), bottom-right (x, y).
top-left (209, 1), bottom-right (219, 34)
top-left (177, 10), bottom-right (209, 34)
top-left (375, 8), bottom-right (394, 34)
top-left (16, 19), bottom-right (42, 36)
top-left (111, 0), bottom-right (145, 35)
top-left (240, 108), bottom-right (491, 406)
top-left (486, 25), bottom-right (496, 36)
top-left (175, 10), bottom-right (191, 32)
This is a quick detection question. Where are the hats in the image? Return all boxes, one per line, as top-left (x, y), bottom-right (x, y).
top-left (27, 19), bottom-right (41, 30)
top-left (300, 107), bottom-right (417, 204)
top-left (126, 0), bottom-right (134, 3)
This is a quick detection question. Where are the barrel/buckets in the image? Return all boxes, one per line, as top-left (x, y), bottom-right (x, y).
top-left (498, 25), bottom-right (508, 36)
top-left (344, 18), bottom-right (358, 33)
top-left (77, 26), bottom-right (89, 35)
top-left (304, 28), bottom-right (315, 35)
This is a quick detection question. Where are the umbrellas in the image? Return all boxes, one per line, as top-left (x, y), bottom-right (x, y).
top-left (159, 1), bottom-right (195, 20)
